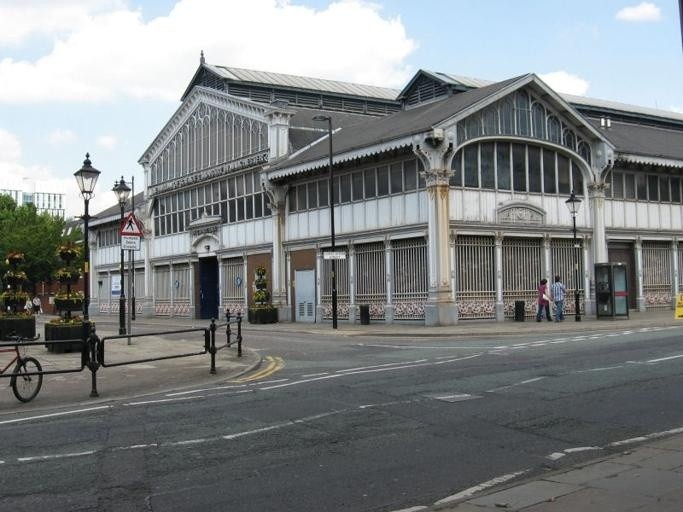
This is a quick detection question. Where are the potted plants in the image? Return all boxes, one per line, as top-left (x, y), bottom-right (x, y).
top-left (247, 265), bottom-right (278, 324)
top-left (1, 244), bottom-right (95, 353)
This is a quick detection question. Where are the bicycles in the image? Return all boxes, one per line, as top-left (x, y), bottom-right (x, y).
top-left (0, 332), bottom-right (43, 402)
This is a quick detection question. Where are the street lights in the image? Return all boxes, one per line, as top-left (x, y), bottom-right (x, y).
top-left (73, 153), bottom-right (101, 366)
top-left (112, 176), bottom-right (131, 335)
top-left (565, 190), bottom-right (582, 321)
top-left (312, 114), bottom-right (339, 328)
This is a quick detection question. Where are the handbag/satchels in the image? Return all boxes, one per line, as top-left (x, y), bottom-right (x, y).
top-left (543, 293), bottom-right (550, 302)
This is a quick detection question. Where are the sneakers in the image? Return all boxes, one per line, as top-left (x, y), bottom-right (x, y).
top-left (536, 317), bottom-right (566, 322)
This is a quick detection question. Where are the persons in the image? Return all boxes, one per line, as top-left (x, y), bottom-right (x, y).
top-left (33, 294), bottom-right (42, 316)
top-left (26, 296), bottom-right (33, 314)
top-left (535, 279), bottom-right (553, 322)
top-left (551, 275), bottom-right (568, 320)
